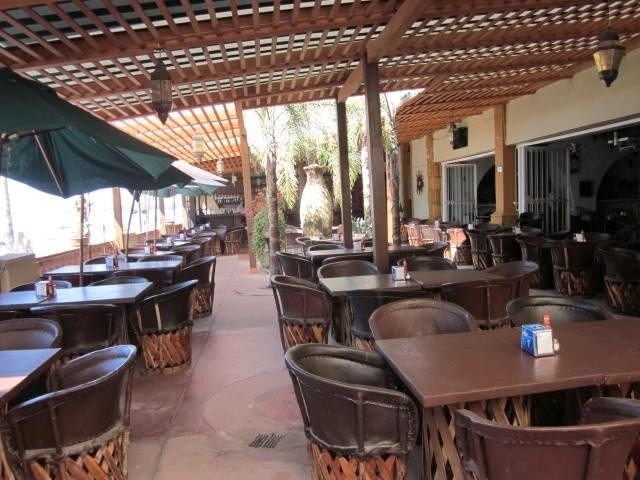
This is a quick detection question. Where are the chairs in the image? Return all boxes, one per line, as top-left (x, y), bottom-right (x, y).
top-left (284, 343), bottom-right (422, 479)
top-left (270, 219), bottom-right (639, 316)
top-left (86, 272), bottom-right (149, 344)
top-left (2, 318), bottom-right (63, 351)
top-left (269, 273), bottom-right (334, 355)
top-left (173, 255), bottom-right (219, 320)
top-left (344, 285), bottom-right (442, 352)
top-left (0, 223), bottom-right (245, 275)
top-left (369, 298), bottom-right (482, 342)
top-left (452, 396), bottom-right (638, 478)
top-left (504, 295), bottom-right (617, 328)
top-left (127, 279), bottom-right (200, 374)
top-left (8, 281), bottom-right (74, 291)
top-left (1, 282), bottom-right (153, 354)
top-left (2, 343), bottom-right (136, 479)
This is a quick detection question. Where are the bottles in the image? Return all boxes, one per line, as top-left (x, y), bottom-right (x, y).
top-left (220, 206), bottom-right (237, 214)
top-left (217, 193), bottom-right (239, 204)
top-left (541, 310), bottom-right (561, 352)
top-left (580, 230), bottom-right (586, 242)
top-left (360, 236), bottom-right (365, 250)
top-left (171, 234), bottom-right (175, 244)
top-left (46, 275), bottom-right (57, 297)
top-left (403, 259), bottom-right (411, 280)
top-left (184, 231), bottom-right (187, 240)
top-left (150, 243), bottom-right (154, 253)
top-left (113, 253), bottom-right (119, 268)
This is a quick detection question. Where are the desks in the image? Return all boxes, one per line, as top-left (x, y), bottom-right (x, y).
top-left (373, 317), bottom-right (640, 480)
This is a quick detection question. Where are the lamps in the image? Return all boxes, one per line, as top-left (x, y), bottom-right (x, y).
top-left (232, 172), bottom-right (237, 187)
top-left (192, 127), bottom-right (204, 164)
top-left (215, 155), bottom-right (225, 178)
top-left (592, 26), bottom-right (626, 86)
top-left (149, 45), bottom-right (171, 127)
